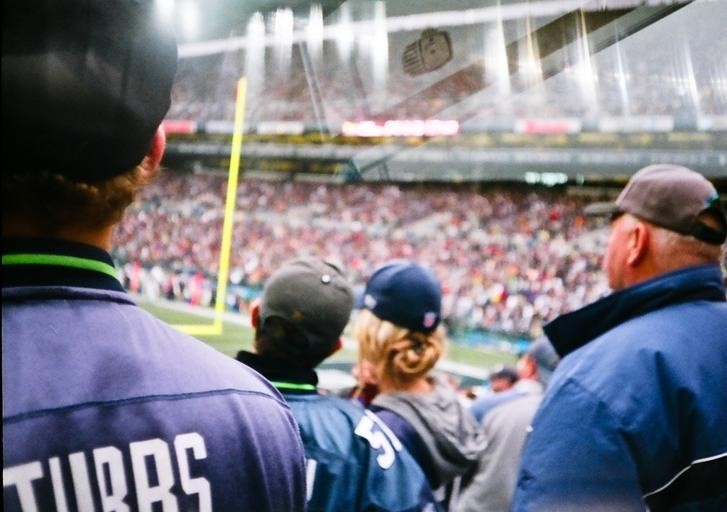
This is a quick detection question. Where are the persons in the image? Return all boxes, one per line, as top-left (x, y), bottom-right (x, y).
top-left (488, 364), bottom-right (518, 394)
top-left (0, 1), bottom-right (308, 512)
top-left (512, 162), bottom-right (726, 511)
top-left (465, 351), bottom-right (545, 420)
top-left (455, 336), bottom-right (560, 512)
top-left (107, 51), bottom-right (725, 340)
top-left (331, 260), bottom-right (486, 512)
top-left (237, 254), bottom-right (442, 510)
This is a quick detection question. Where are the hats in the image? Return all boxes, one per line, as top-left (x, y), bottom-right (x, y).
top-left (583, 164), bottom-right (726, 244)
top-left (0, 1), bottom-right (176, 182)
top-left (259, 256), bottom-right (353, 349)
top-left (358, 260), bottom-right (441, 334)
